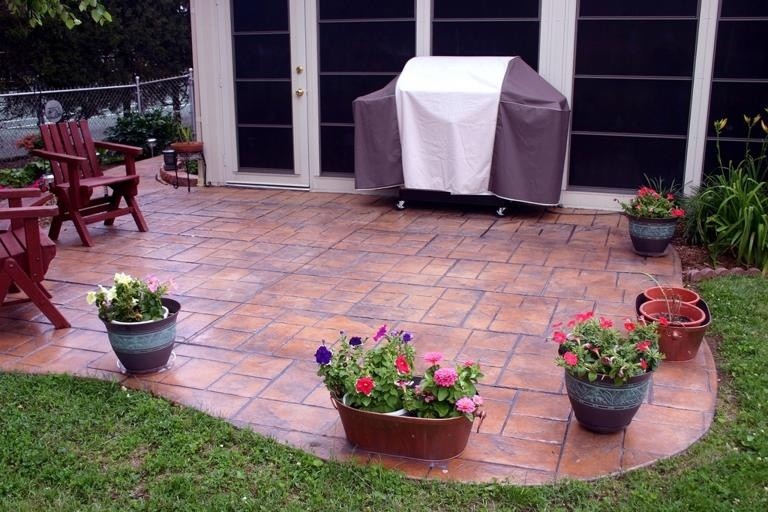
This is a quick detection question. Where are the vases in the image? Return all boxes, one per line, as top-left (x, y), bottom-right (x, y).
top-left (330, 375), bottom-right (475, 462)
top-left (629, 217), bottom-right (678, 255)
top-left (97, 298), bottom-right (181, 372)
top-left (643, 285), bottom-right (700, 306)
top-left (563, 367), bottom-right (655, 434)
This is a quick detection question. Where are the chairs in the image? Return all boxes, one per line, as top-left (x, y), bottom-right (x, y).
top-left (0, 187), bottom-right (71, 330)
top-left (30, 118), bottom-right (147, 248)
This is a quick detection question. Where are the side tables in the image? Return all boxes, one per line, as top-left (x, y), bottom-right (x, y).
top-left (1, 191), bottom-right (53, 233)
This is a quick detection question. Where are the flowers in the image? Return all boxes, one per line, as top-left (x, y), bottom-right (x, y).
top-left (86, 272), bottom-right (172, 321)
top-left (547, 309), bottom-right (668, 383)
top-left (616, 187), bottom-right (685, 219)
top-left (314, 325), bottom-right (486, 417)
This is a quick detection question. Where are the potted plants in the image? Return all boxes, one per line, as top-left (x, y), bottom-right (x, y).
top-left (637, 269), bottom-right (707, 326)
top-left (170, 121), bottom-right (204, 153)
